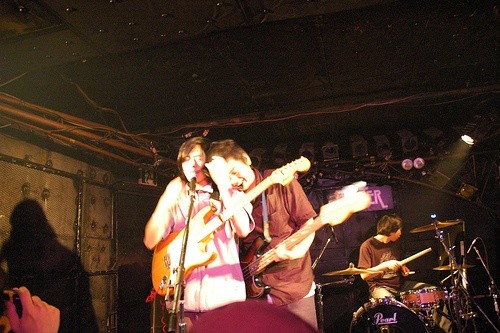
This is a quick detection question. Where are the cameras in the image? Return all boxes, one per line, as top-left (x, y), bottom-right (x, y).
top-left (0, 292), bottom-right (22, 318)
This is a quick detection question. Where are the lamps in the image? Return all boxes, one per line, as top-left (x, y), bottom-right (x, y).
top-left (350, 136), bottom-right (368, 159)
top-left (153, 140), bottom-right (179, 166)
top-left (321, 139), bottom-right (342, 162)
top-left (299, 144), bottom-right (316, 161)
top-left (373, 116), bottom-right (493, 170)
top-left (249, 148), bottom-right (267, 169)
top-left (271, 143), bottom-right (294, 162)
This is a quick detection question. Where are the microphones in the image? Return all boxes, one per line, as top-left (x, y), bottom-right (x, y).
top-left (467, 239), bottom-right (477, 253)
top-left (190, 173), bottom-right (199, 192)
top-left (331, 226), bottom-right (339, 244)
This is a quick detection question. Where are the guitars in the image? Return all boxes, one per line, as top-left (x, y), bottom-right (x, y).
top-left (242, 180), bottom-right (373, 299)
top-left (152, 154), bottom-right (312, 296)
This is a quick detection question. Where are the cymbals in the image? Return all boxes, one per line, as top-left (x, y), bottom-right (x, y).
top-left (409, 219), bottom-right (463, 233)
top-left (320, 267), bottom-right (383, 275)
top-left (432, 263), bottom-right (476, 270)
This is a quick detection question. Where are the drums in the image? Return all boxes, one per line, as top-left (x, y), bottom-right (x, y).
top-left (399, 286), bottom-right (445, 308)
top-left (349, 297), bottom-right (430, 333)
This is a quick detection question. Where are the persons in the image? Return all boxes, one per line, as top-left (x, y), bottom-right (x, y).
top-left (357, 214), bottom-right (454, 333)
top-left (205, 139), bottom-right (317, 332)
top-left (0, 286), bottom-right (60, 333)
top-left (143, 137), bottom-right (255, 333)
